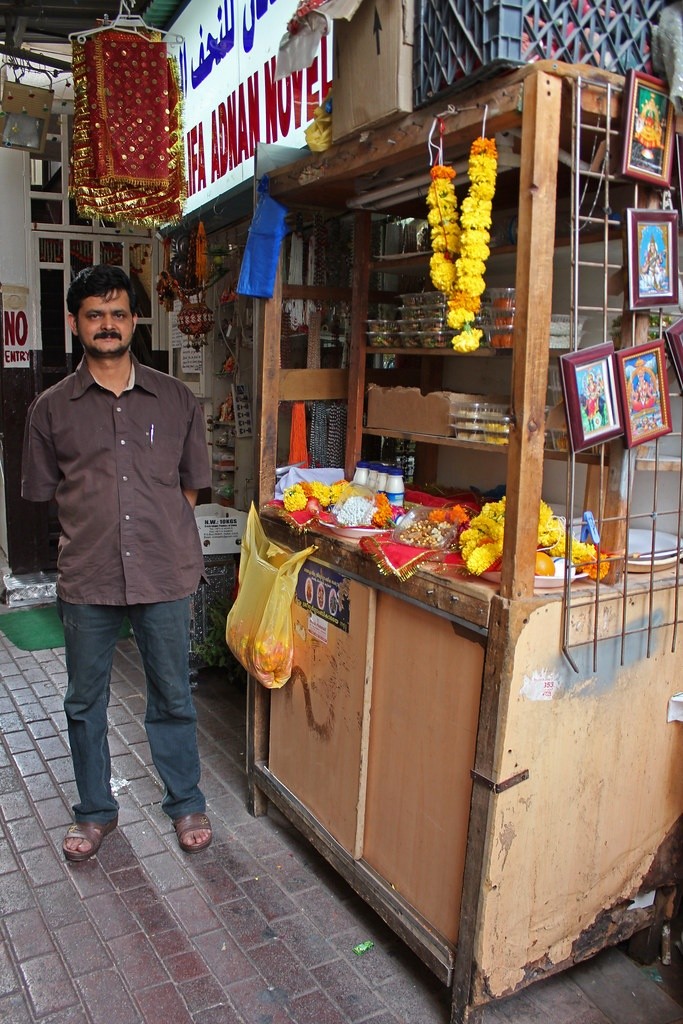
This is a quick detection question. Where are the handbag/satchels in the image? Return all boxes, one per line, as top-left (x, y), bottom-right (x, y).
top-left (233, 192), bottom-right (289, 298)
top-left (224, 499), bottom-right (320, 691)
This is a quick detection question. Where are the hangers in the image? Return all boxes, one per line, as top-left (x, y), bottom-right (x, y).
top-left (67, 0), bottom-right (185, 49)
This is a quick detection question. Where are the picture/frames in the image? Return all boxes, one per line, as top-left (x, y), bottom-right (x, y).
top-left (558, 340), bottom-right (625, 455)
top-left (622, 207), bottom-right (678, 310)
top-left (665, 316), bottom-right (683, 389)
top-left (618, 68), bottom-right (676, 189)
top-left (614, 339), bottom-right (673, 449)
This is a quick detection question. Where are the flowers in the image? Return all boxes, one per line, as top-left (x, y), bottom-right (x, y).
top-left (457, 495), bottom-right (610, 577)
top-left (427, 136), bottom-right (498, 355)
top-left (285, 479), bottom-right (401, 529)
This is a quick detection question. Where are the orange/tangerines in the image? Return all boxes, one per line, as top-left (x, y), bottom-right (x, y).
top-left (535, 552), bottom-right (555, 577)
top-left (490, 298), bottom-right (514, 347)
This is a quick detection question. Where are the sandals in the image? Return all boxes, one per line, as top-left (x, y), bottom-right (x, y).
top-left (61, 815), bottom-right (118, 861)
top-left (173, 812), bottom-right (212, 853)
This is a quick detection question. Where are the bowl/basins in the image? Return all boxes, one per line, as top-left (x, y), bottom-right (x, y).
top-left (364, 287), bottom-right (590, 350)
top-left (448, 397), bottom-right (596, 455)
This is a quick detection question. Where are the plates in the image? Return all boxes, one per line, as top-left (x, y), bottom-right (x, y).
top-left (623, 529), bottom-right (683, 573)
top-left (317, 517), bottom-right (395, 540)
top-left (475, 568), bottom-right (590, 589)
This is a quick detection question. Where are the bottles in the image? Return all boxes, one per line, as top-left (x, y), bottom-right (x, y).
top-left (220, 451), bottom-right (234, 471)
top-left (547, 556), bottom-right (576, 577)
top-left (352, 461), bottom-right (405, 508)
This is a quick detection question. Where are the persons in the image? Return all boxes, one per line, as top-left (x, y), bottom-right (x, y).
top-left (19, 264), bottom-right (215, 864)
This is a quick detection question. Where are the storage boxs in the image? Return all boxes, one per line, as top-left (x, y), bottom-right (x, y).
top-left (193, 503), bottom-right (249, 555)
top-left (267, 0), bottom-right (419, 145)
top-left (368, 383), bottom-right (510, 436)
top-left (414, 0), bottom-right (659, 110)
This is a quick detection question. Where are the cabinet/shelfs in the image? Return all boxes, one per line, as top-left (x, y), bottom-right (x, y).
top-left (209, 278), bottom-right (238, 509)
top-left (244, 58), bottom-right (683, 1024)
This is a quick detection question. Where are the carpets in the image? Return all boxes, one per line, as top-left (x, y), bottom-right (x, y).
top-left (0, 603), bottom-right (137, 651)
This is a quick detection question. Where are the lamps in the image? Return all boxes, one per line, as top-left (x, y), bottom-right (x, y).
top-left (0, 62), bottom-right (54, 155)
